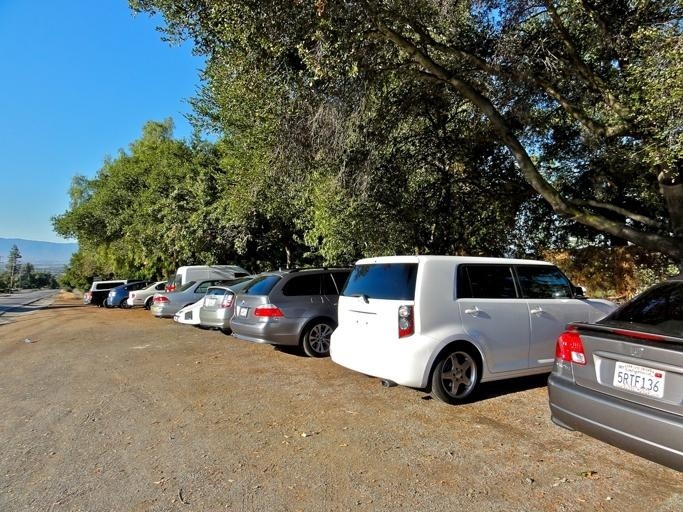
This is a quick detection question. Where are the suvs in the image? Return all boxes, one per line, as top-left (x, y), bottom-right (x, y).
top-left (328, 252), bottom-right (623, 406)
top-left (228, 262), bottom-right (354, 358)
top-left (82, 279), bottom-right (142, 309)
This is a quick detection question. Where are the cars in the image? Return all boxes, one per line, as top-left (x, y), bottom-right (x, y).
top-left (546, 274), bottom-right (683, 476)
top-left (105, 280), bottom-right (169, 310)
top-left (150, 271), bottom-right (267, 335)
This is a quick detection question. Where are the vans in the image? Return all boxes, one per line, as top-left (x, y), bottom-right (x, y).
top-left (174, 264), bottom-right (252, 291)
